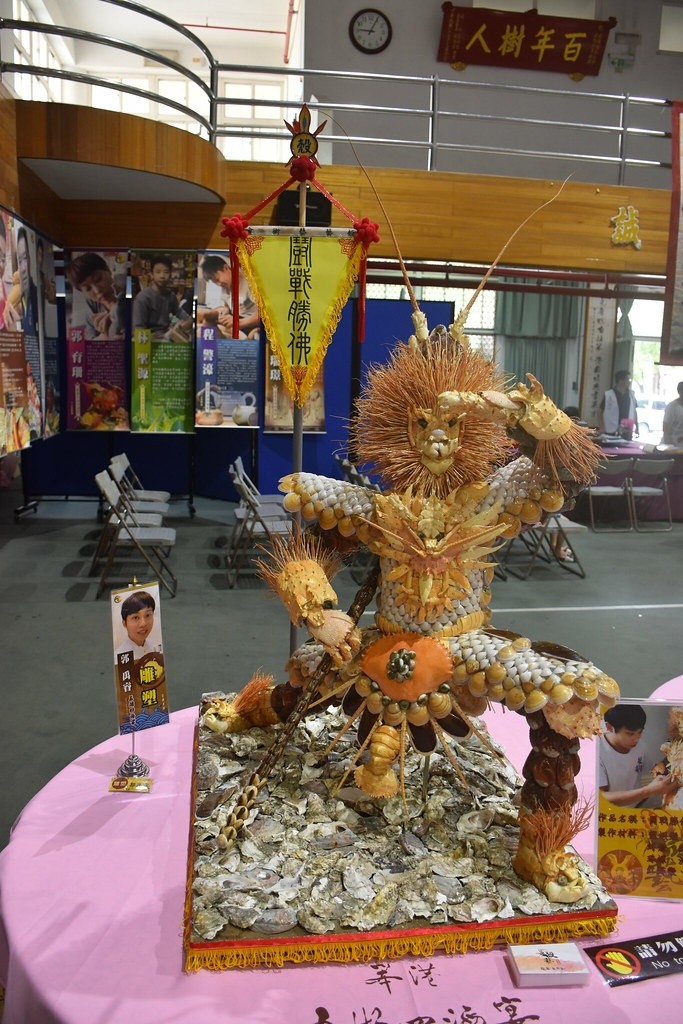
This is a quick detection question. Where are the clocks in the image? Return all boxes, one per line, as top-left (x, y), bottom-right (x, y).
top-left (348, 8), bottom-right (393, 54)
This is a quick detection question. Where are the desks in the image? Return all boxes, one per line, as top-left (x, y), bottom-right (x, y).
top-left (574, 440), bottom-right (683, 522)
top-left (0, 702), bottom-right (683, 1024)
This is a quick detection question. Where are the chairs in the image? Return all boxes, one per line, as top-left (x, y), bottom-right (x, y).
top-left (227, 477), bottom-right (303, 591)
top-left (89, 470), bottom-right (176, 575)
top-left (99, 461), bottom-right (172, 558)
top-left (607, 457), bottom-right (676, 533)
top-left (234, 456), bottom-right (285, 509)
top-left (572, 457), bottom-right (634, 533)
top-left (223, 464), bottom-right (288, 569)
top-left (488, 512), bottom-right (588, 580)
top-left (111, 453), bottom-right (171, 503)
top-left (98, 481), bottom-right (178, 599)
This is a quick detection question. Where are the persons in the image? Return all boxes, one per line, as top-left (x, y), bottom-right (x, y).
top-left (0, 215), bottom-right (261, 341)
top-left (598, 371), bottom-right (640, 441)
top-left (598, 704), bottom-right (678, 807)
top-left (660, 381), bottom-right (683, 446)
top-left (114, 591), bottom-right (162, 665)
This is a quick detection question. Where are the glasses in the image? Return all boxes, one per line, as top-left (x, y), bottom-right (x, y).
top-left (625, 379), bottom-right (632, 382)
